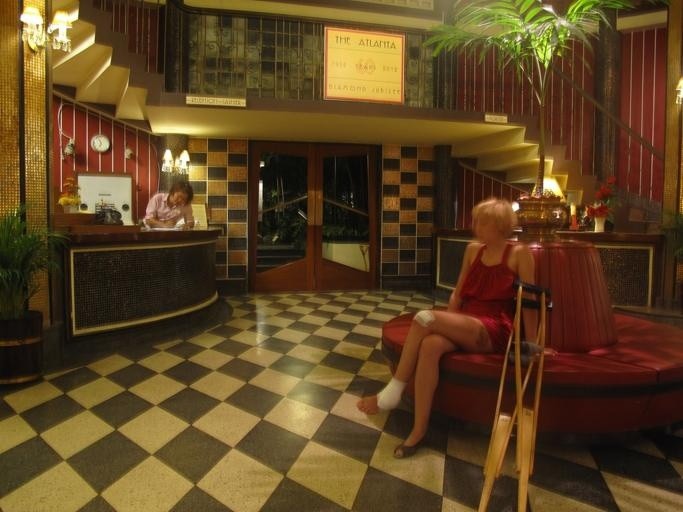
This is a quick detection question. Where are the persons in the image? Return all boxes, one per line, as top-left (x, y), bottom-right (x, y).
top-left (357, 198), bottom-right (559, 458)
top-left (141, 179), bottom-right (193, 233)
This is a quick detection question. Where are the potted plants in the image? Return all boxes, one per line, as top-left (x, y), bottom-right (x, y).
top-left (423, 0), bottom-right (635, 242)
top-left (0, 201), bottom-right (71, 390)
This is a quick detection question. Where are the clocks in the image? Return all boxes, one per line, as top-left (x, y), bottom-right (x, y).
top-left (92, 135), bottom-right (109, 152)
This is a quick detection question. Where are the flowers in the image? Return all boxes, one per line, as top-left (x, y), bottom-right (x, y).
top-left (604, 176), bottom-right (624, 223)
top-left (587, 184), bottom-right (612, 218)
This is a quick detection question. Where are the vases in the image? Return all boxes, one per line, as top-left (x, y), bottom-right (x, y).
top-left (594, 217), bottom-right (606, 233)
top-left (603, 219), bottom-right (613, 232)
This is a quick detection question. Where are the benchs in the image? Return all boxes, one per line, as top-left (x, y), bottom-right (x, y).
top-left (382, 237), bottom-right (683, 432)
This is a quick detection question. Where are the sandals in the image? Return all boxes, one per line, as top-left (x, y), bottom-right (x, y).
top-left (392, 425), bottom-right (432, 459)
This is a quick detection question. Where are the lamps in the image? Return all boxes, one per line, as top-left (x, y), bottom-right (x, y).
top-left (62, 133), bottom-right (76, 155)
top-left (161, 148), bottom-right (191, 175)
top-left (20, 6), bottom-right (73, 53)
